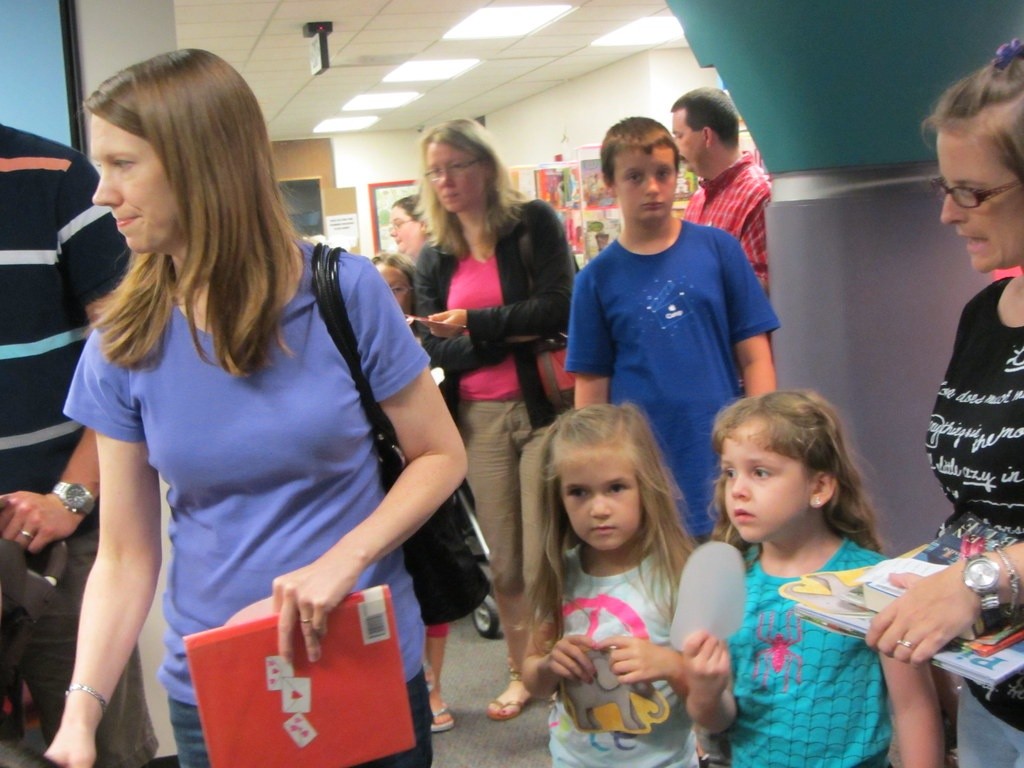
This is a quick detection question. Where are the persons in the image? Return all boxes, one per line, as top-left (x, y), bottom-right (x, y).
top-left (521, 402), bottom-right (701, 768)
top-left (390, 194), bottom-right (431, 267)
top-left (682, 389), bottom-right (945, 768)
top-left (0, 123), bottom-right (159, 768)
top-left (369, 253), bottom-right (454, 732)
top-left (44, 48), bottom-right (469, 768)
top-left (671, 86), bottom-right (772, 297)
top-left (564, 118), bottom-right (782, 544)
top-left (413, 118), bottom-right (581, 721)
top-left (866, 39), bottom-right (1024, 768)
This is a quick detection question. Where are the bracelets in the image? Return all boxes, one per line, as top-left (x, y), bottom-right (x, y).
top-left (65, 683), bottom-right (108, 718)
top-left (993, 546), bottom-right (1020, 616)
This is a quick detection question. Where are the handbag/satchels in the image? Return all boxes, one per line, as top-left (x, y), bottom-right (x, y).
top-left (536, 331), bottom-right (577, 412)
top-left (311, 240), bottom-right (491, 626)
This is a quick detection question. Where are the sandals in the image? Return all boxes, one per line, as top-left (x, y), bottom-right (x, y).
top-left (485, 671), bottom-right (533, 720)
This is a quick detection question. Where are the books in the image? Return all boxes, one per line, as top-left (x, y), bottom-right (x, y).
top-left (182, 584), bottom-right (416, 768)
top-left (794, 512), bottom-right (1024, 691)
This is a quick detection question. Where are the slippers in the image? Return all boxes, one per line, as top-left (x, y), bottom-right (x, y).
top-left (425, 683), bottom-right (454, 733)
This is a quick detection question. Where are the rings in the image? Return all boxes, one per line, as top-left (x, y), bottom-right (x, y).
top-left (300, 619), bottom-right (313, 623)
top-left (21, 531), bottom-right (34, 539)
top-left (897, 640), bottom-right (916, 650)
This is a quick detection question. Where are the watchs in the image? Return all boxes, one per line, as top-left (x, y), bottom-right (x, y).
top-left (961, 553), bottom-right (1001, 612)
top-left (52, 481), bottom-right (95, 515)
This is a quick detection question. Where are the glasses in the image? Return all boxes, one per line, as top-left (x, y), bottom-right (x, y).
top-left (388, 218), bottom-right (415, 233)
top-left (931, 176), bottom-right (1021, 208)
top-left (423, 157), bottom-right (483, 182)
